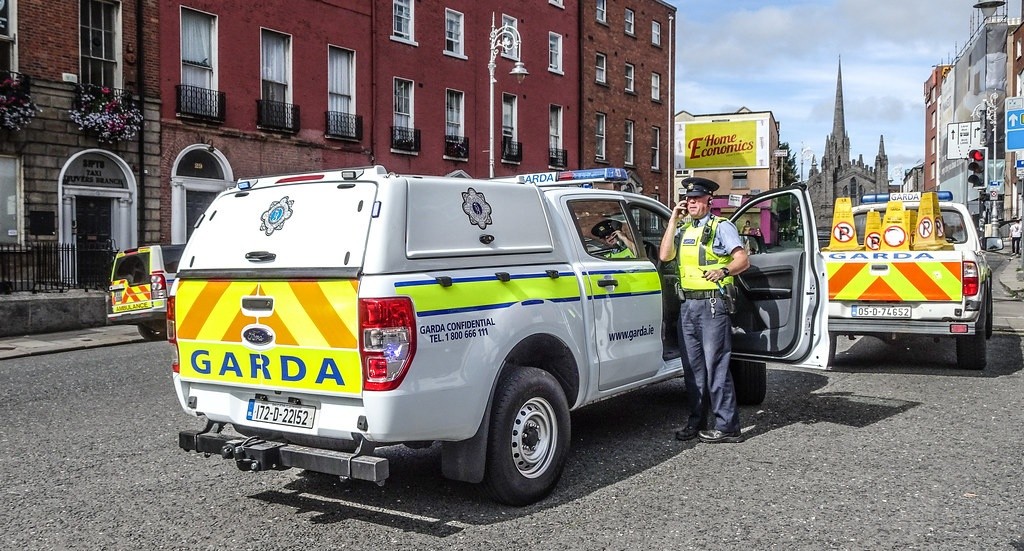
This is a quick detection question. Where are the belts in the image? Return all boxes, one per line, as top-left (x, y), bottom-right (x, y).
top-left (683, 290), bottom-right (723, 299)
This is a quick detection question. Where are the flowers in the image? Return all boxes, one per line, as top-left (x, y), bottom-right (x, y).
top-left (0, 72), bottom-right (44, 131)
top-left (69, 84), bottom-right (144, 144)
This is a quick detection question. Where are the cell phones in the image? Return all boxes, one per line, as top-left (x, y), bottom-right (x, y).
top-left (682, 204), bottom-right (688, 216)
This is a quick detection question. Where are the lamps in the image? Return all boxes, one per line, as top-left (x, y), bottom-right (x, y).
top-left (201, 136), bottom-right (215, 152)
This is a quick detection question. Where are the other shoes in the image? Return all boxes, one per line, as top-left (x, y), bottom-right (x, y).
top-left (1011, 253), bottom-right (1015, 256)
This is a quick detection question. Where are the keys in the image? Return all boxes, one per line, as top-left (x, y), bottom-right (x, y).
top-left (710, 304), bottom-right (716, 320)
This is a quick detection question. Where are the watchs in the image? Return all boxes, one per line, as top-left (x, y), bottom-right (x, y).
top-left (721, 267), bottom-right (730, 277)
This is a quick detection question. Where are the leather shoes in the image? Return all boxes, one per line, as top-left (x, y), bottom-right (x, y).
top-left (677, 426), bottom-right (708, 440)
top-left (698, 428), bottom-right (744, 444)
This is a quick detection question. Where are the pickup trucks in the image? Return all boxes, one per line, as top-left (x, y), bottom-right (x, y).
top-left (816, 191), bottom-right (994, 368)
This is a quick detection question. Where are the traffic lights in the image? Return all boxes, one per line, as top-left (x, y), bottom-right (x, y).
top-left (965, 147), bottom-right (988, 189)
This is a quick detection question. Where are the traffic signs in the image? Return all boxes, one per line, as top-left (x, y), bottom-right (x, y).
top-left (1005, 96), bottom-right (1024, 152)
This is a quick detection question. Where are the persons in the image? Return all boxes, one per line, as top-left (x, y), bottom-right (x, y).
top-left (660, 177), bottom-right (751, 443)
top-left (1009, 220), bottom-right (1023, 256)
top-left (590, 218), bottom-right (638, 259)
top-left (741, 220), bottom-right (751, 255)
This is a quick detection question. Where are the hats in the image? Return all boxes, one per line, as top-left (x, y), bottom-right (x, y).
top-left (590, 219), bottom-right (623, 238)
top-left (681, 177), bottom-right (720, 196)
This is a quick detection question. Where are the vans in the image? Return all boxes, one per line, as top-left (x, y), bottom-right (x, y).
top-left (107, 243), bottom-right (192, 342)
top-left (163, 160), bottom-right (831, 509)
